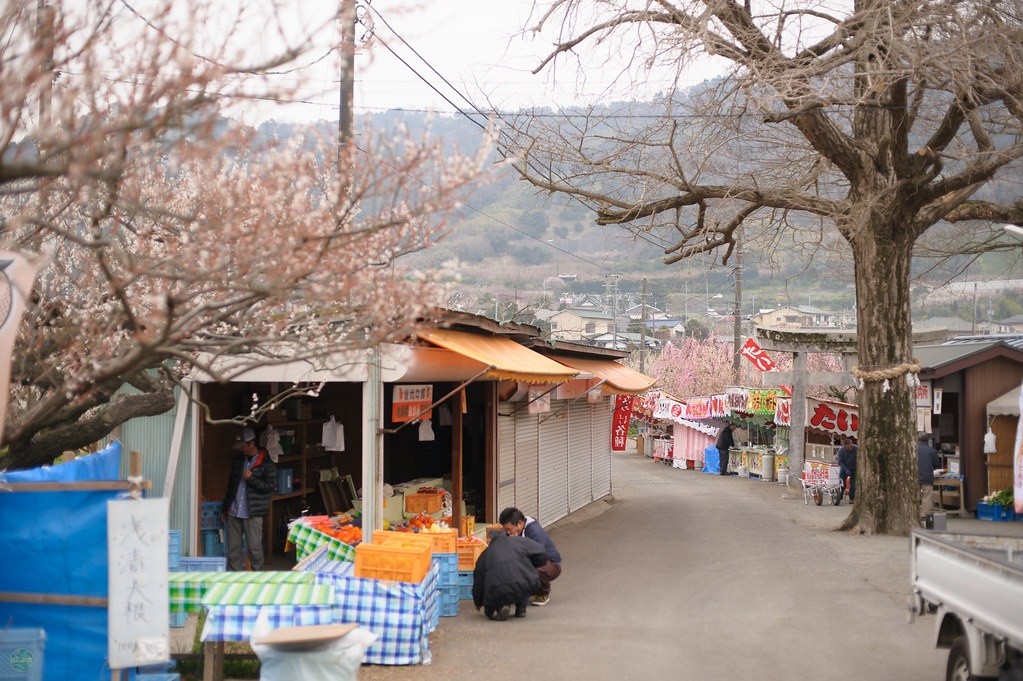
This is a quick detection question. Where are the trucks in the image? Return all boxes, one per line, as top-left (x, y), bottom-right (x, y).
top-left (906, 528), bottom-right (1023, 681)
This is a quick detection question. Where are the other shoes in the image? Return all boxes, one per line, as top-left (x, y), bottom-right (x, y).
top-left (495, 606), bottom-right (510, 621)
top-left (839, 495), bottom-right (843, 501)
top-left (719, 472), bottom-right (730, 475)
top-left (514, 613), bottom-right (526, 617)
top-left (849, 499), bottom-right (854, 504)
top-left (531, 596), bottom-right (550, 606)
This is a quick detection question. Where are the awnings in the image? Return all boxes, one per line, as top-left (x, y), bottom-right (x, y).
top-left (540, 355), bottom-right (659, 425)
top-left (384, 327), bottom-right (580, 433)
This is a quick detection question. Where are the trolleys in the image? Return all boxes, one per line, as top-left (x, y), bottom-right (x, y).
top-left (796, 477), bottom-right (845, 506)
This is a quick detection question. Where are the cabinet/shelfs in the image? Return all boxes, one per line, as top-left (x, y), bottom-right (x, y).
top-left (247, 417), bottom-right (329, 560)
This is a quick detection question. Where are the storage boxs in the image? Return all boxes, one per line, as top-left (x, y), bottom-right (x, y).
top-left (200, 502), bottom-right (223, 530)
top-left (169, 529), bottom-right (182, 572)
top-left (978, 500), bottom-right (1015, 520)
top-left (287, 399), bottom-right (302, 420)
top-left (278, 468), bottom-right (293, 495)
top-left (177, 557), bottom-right (227, 572)
top-left (278, 430), bottom-right (295, 456)
top-left (350, 494), bottom-right (500, 616)
top-left (169, 610), bottom-right (188, 627)
top-left (302, 403), bottom-right (312, 420)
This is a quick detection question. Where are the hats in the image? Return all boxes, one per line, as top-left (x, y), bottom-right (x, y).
top-left (233, 430), bottom-right (255, 449)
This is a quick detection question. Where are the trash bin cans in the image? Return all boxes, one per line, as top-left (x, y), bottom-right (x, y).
top-left (637, 435), bottom-right (644, 455)
top-left (761, 455), bottom-right (773, 482)
top-left (926, 510), bottom-right (947, 530)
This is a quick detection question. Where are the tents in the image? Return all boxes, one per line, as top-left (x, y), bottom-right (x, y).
top-left (654, 385), bottom-right (859, 478)
top-left (987, 383), bottom-right (1023, 496)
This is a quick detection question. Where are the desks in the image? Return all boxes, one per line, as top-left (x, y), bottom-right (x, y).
top-left (167, 570), bottom-right (318, 661)
top-left (199, 582), bottom-right (334, 681)
top-left (311, 557), bottom-right (439, 665)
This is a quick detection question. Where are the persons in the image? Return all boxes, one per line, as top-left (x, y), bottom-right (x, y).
top-left (472, 523), bottom-right (547, 620)
top-left (499, 508), bottom-right (561, 605)
top-left (836, 439), bottom-right (858, 503)
top-left (220, 427), bottom-right (278, 570)
top-left (716, 423), bottom-right (737, 474)
top-left (918, 430), bottom-right (939, 517)
